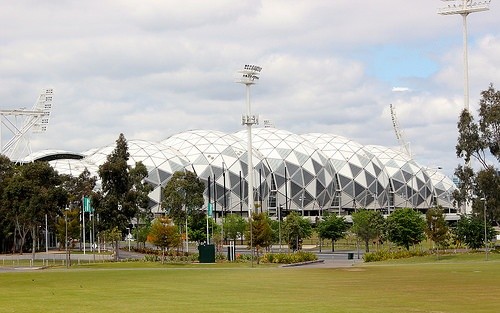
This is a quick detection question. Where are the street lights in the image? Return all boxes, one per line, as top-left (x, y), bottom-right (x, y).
top-left (65, 208), bottom-right (70, 268)
top-left (235, 65), bottom-right (262, 217)
top-left (480, 198), bottom-right (487, 260)
top-left (437, 0), bottom-right (491, 214)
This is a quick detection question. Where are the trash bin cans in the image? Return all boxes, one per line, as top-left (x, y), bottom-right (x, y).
top-left (198, 244), bottom-right (215, 263)
top-left (348, 253), bottom-right (354, 260)
